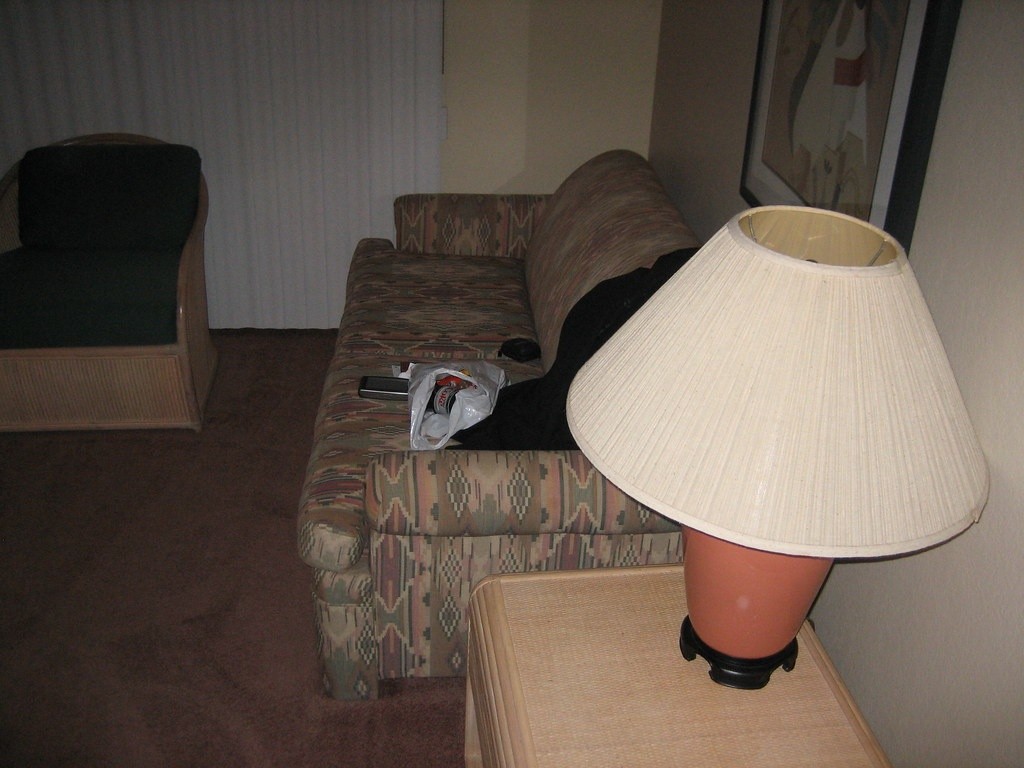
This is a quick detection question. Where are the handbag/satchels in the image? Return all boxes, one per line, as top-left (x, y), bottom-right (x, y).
top-left (500, 337), bottom-right (541, 363)
top-left (407, 357), bottom-right (513, 451)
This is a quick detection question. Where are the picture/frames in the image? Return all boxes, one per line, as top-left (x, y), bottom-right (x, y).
top-left (739, 0), bottom-right (963, 262)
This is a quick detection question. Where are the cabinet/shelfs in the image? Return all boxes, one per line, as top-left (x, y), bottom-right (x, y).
top-left (464, 564), bottom-right (892, 768)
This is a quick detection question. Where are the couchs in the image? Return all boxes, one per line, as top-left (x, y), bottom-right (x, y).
top-left (297, 150), bottom-right (701, 702)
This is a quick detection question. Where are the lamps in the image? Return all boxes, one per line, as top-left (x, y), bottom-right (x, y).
top-left (566, 204), bottom-right (992, 690)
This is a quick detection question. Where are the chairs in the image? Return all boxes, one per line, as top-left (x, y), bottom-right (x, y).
top-left (0, 132), bottom-right (219, 434)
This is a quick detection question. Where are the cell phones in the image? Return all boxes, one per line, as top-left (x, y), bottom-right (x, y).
top-left (358, 376), bottom-right (410, 400)
